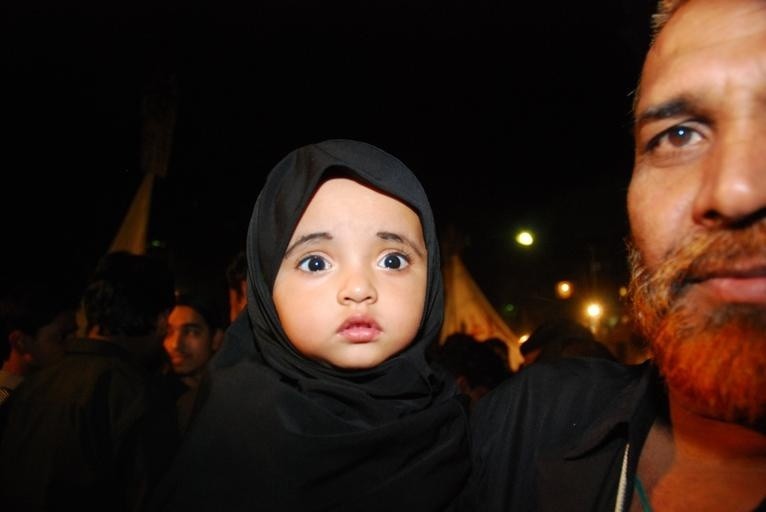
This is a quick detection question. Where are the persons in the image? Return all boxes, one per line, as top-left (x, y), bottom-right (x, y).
top-left (434, 331), bottom-right (510, 416)
top-left (519, 317), bottom-right (596, 368)
top-left (0, 291), bottom-right (73, 408)
top-left (136, 138), bottom-right (673, 511)
top-left (614, 334), bottom-right (658, 367)
top-left (2, 246), bottom-right (196, 511)
top-left (605, 0), bottom-right (766, 511)
top-left (159, 269), bottom-right (232, 404)
